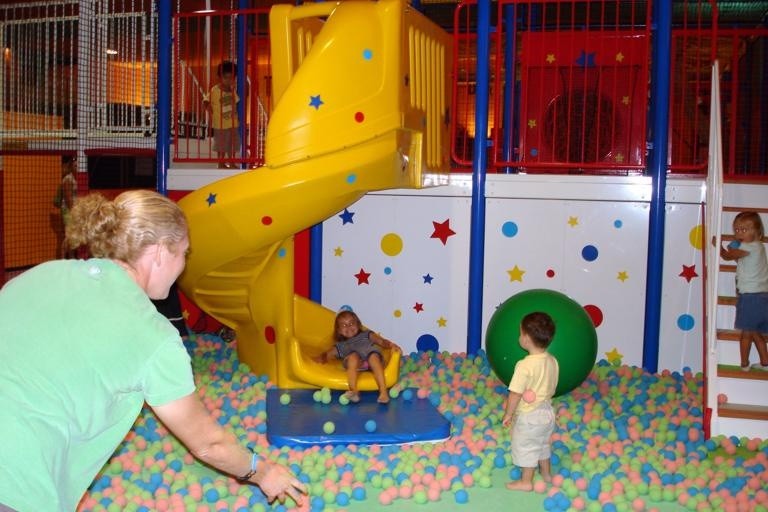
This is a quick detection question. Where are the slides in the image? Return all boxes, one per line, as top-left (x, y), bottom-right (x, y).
top-left (176, 0), bottom-right (452, 391)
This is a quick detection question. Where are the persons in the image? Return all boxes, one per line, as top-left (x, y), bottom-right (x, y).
top-left (712, 211), bottom-right (767, 373)
top-left (1, 186), bottom-right (313, 512)
top-left (202, 62), bottom-right (244, 168)
top-left (311, 312), bottom-right (401, 404)
top-left (503, 313), bottom-right (560, 492)
